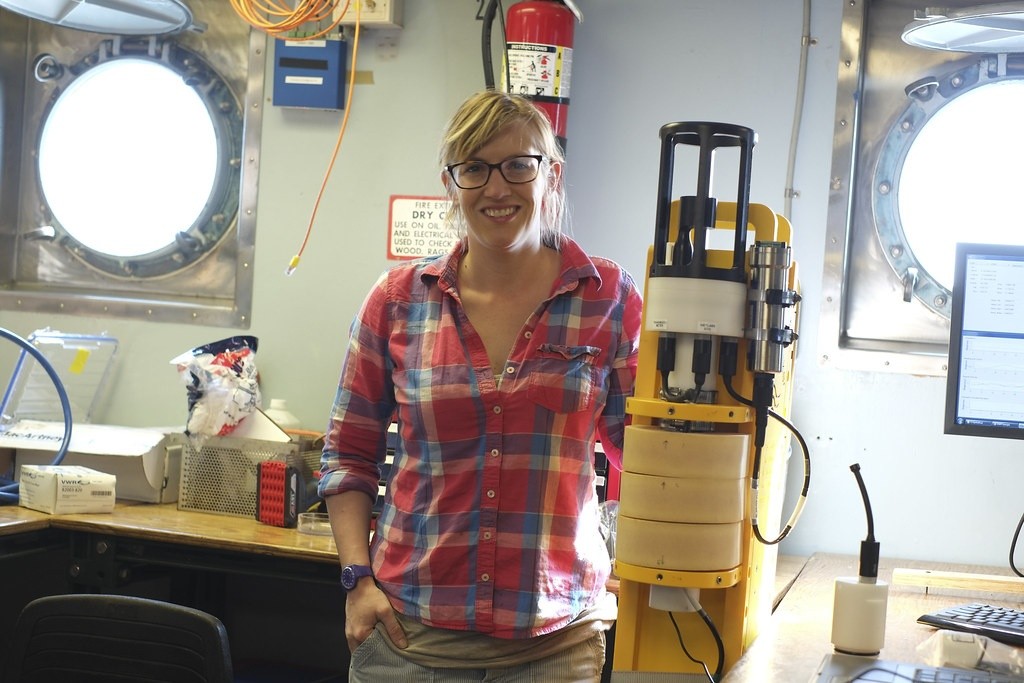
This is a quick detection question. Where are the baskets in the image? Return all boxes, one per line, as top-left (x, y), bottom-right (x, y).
top-left (177, 432), bottom-right (324, 521)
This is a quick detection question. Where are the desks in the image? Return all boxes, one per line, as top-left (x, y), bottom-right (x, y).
top-left (0, 496), bottom-right (342, 589)
top-left (721, 550), bottom-right (1024, 683)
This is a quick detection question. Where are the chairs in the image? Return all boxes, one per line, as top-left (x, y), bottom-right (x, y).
top-left (16, 593), bottom-right (235, 682)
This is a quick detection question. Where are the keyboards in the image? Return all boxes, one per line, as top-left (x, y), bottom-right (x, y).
top-left (917, 602), bottom-right (1024, 647)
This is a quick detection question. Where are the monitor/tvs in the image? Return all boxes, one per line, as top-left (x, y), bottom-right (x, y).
top-left (944, 243), bottom-right (1023, 441)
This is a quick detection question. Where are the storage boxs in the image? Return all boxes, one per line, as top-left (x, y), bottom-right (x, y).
top-left (19, 463), bottom-right (117, 515)
top-left (0, 418), bottom-right (186, 503)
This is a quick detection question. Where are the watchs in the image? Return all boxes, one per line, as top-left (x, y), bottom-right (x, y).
top-left (341, 564), bottom-right (374, 590)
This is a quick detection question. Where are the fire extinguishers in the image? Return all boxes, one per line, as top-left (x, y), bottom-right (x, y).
top-left (499, 0), bottom-right (576, 209)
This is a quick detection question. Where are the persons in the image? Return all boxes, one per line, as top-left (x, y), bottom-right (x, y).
top-left (317, 91), bottom-right (644, 683)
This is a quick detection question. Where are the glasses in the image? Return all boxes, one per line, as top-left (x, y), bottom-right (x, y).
top-left (445, 155), bottom-right (550, 189)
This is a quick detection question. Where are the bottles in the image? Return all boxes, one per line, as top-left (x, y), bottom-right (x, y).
top-left (244, 398), bottom-right (300, 493)
